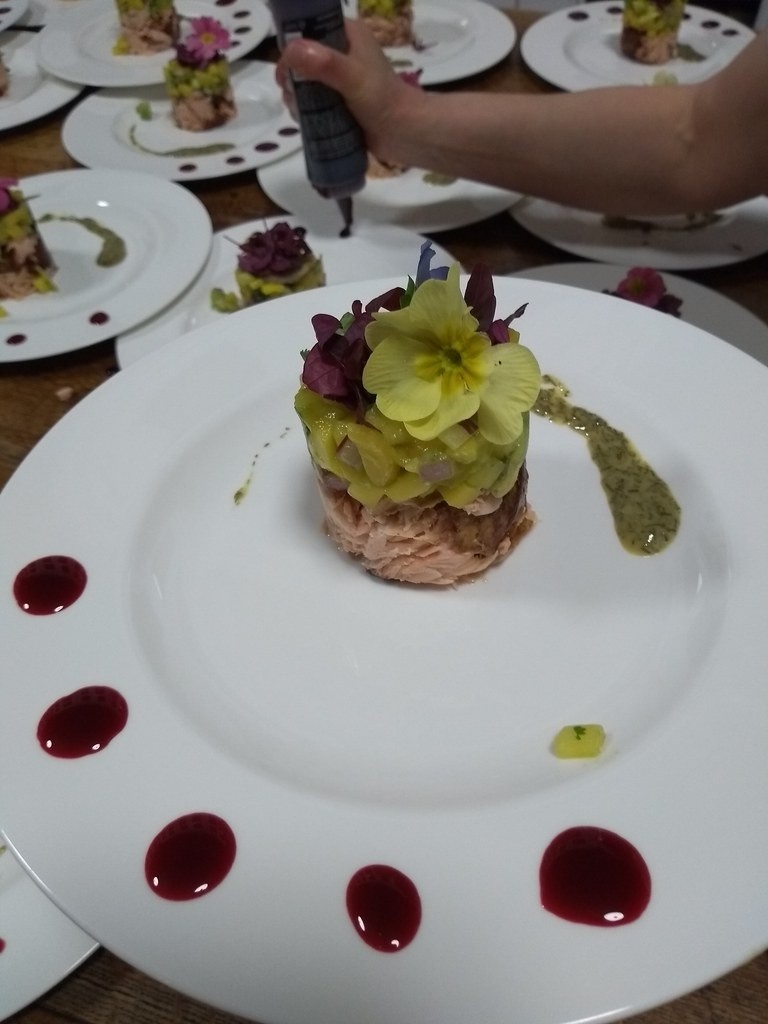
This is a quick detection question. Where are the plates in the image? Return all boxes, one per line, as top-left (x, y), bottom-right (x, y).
top-left (0, 28), bottom-right (85, 129)
top-left (506, 263), bottom-right (767, 368)
top-left (30, 0), bottom-right (272, 87)
top-left (62, 59), bottom-right (303, 181)
top-left (519, 1), bottom-right (757, 97)
top-left (0, 166), bottom-right (212, 361)
top-left (16, 0), bottom-right (81, 26)
top-left (0, 0), bottom-right (29, 31)
top-left (505, 196), bottom-right (768, 270)
top-left (114, 215), bottom-right (465, 367)
top-left (256, 146), bottom-right (527, 232)
top-left (340, 1), bottom-right (517, 87)
top-left (0, 833), bottom-right (100, 1022)
top-left (0, 274), bottom-right (768, 1024)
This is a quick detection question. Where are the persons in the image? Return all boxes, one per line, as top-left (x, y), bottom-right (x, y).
top-left (276, 1), bottom-right (767, 220)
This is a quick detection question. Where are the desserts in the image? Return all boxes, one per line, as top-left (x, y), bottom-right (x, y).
top-left (0, 0), bottom-right (716, 584)
